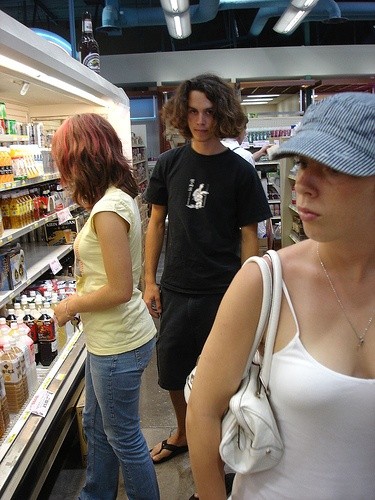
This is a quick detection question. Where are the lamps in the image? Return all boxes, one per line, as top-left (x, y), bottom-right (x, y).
top-left (272, 0), bottom-right (320, 35)
top-left (161, 0), bottom-right (192, 39)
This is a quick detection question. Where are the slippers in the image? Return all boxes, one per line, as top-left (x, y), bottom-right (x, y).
top-left (188, 473), bottom-right (238, 500)
top-left (148, 439), bottom-right (188, 465)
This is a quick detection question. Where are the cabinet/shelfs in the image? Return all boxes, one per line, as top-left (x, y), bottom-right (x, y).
top-left (241, 132), bottom-right (290, 251)
top-left (132, 134), bottom-right (151, 218)
top-left (0, 11), bottom-right (132, 500)
top-left (279, 138), bottom-right (308, 248)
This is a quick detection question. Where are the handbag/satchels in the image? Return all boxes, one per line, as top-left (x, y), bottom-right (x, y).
top-left (183, 249), bottom-right (285, 473)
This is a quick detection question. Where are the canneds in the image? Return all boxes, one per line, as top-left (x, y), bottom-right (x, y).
top-left (248, 129), bottom-right (291, 143)
top-left (0, 102), bottom-right (17, 134)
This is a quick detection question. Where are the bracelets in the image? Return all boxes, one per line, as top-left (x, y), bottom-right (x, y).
top-left (65, 301), bottom-right (76, 318)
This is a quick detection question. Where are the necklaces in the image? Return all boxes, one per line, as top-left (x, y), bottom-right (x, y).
top-left (317, 242), bottom-right (375, 347)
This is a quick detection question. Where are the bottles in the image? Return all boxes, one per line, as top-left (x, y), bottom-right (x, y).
top-left (79, 10), bottom-right (100, 76)
top-left (0, 278), bottom-right (80, 438)
top-left (0, 102), bottom-right (75, 229)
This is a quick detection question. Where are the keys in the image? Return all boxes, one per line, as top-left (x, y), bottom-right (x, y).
top-left (71, 317), bottom-right (80, 332)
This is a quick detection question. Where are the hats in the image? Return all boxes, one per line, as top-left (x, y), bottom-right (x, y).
top-left (271, 91), bottom-right (375, 177)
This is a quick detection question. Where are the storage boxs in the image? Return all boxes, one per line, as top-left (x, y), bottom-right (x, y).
top-left (135, 193), bottom-right (150, 296)
top-left (258, 237), bottom-right (269, 257)
top-left (75, 386), bottom-right (89, 468)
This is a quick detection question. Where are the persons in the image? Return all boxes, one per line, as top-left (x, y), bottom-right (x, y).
top-left (218, 115), bottom-right (274, 169)
top-left (143, 74), bottom-right (273, 500)
top-left (185, 91), bottom-right (374, 500)
top-left (51, 113), bottom-right (163, 500)
